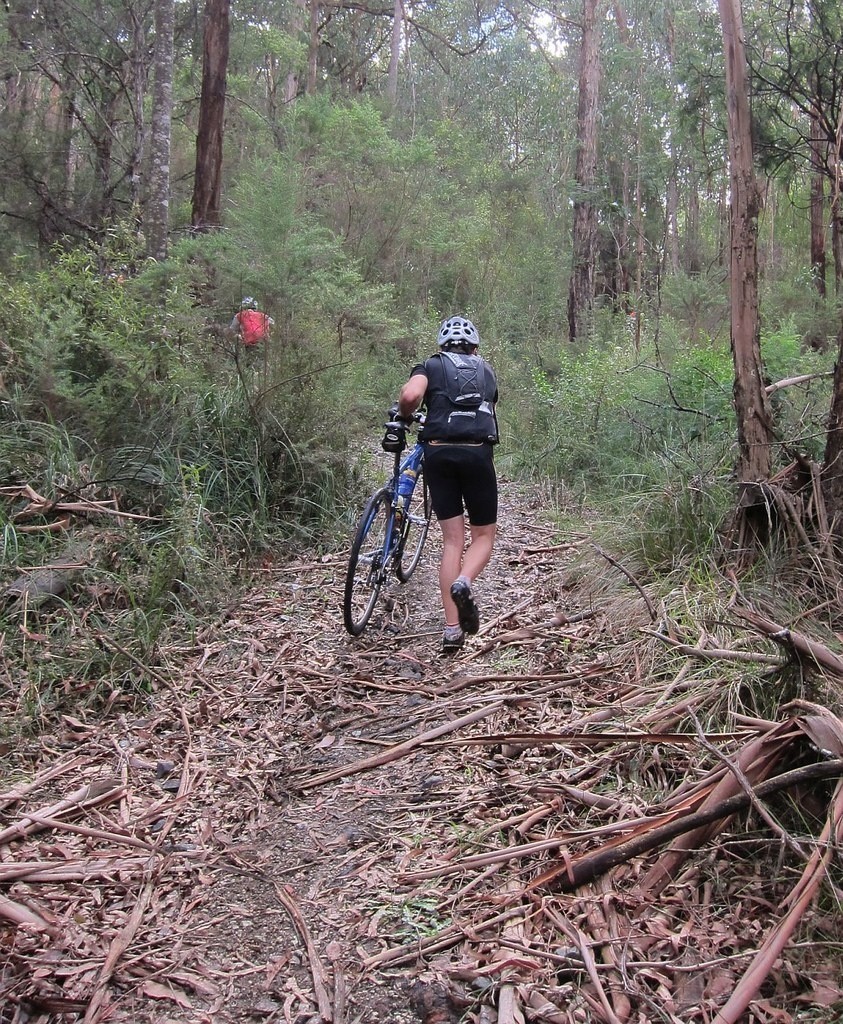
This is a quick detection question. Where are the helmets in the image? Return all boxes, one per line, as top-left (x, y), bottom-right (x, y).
top-left (437, 316), bottom-right (479, 346)
top-left (242, 296), bottom-right (259, 311)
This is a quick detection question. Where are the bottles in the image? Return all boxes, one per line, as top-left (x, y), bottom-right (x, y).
top-left (392, 494), bottom-right (403, 538)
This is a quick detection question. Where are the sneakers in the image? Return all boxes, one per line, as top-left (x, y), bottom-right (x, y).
top-left (450, 580), bottom-right (479, 635)
top-left (443, 632), bottom-right (465, 650)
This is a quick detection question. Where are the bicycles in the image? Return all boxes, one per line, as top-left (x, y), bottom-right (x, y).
top-left (343, 399), bottom-right (433, 637)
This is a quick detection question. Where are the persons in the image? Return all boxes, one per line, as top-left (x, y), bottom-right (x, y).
top-left (223, 296), bottom-right (277, 396)
top-left (394, 317), bottom-right (499, 650)
top-left (107, 264), bottom-right (127, 289)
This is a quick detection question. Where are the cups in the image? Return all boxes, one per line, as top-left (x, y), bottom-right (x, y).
top-left (398, 470), bottom-right (416, 496)
